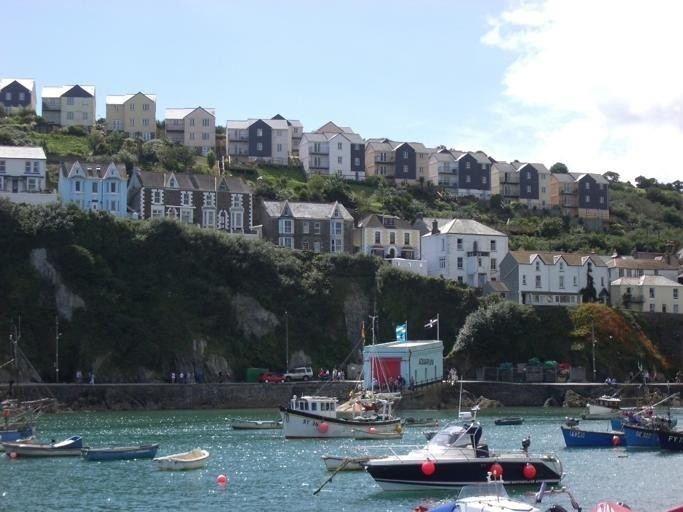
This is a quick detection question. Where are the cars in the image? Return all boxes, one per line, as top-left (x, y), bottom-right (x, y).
top-left (257, 371), bottom-right (286, 385)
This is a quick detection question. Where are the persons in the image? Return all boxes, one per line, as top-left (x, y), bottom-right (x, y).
top-left (675, 370), bottom-right (683, 384)
top-left (523, 436), bottom-right (527, 441)
top-left (75, 367), bottom-right (96, 385)
top-left (604, 375), bottom-right (612, 383)
top-left (447, 366), bottom-right (457, 381)
top-left (386, 375), bottom-right (402, 391)
top-left (167, 372), bottom-right (185, 383)
top-left (644, 369), bottom-right (649, 382)
top-left (522, 435), bottom-right (530, 457)
top-left (319, 367), bottom-right (345, 382)
top-left (612, 376), bottom-right (617, 383)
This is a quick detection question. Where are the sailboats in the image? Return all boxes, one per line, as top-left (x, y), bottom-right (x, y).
top-left (277, 292), bottom-right (409, 440)
top-left (0, 313), bottom-right (58, 450)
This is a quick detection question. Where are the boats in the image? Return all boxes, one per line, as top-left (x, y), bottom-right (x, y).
top-left (410, 471), bottom-right (683, 512)
top-left (229, 417), bottom-right (284, 431)
top-left (560, 360), bottom-right (683, 455)
top-left (4, 435), bottom-right (84, 458)
top-left (405, 415), bottom-right (440, 427)
top-left (321, 453), bottom-right (388, 472)
top-left (152, 445), bottom-right (211, 472)
top-left (79, 442), bottom-right (160, 461)
top-left (494, 414), bottom-right (525, 425)
top-left (352, 374), bottom-right (568, 493)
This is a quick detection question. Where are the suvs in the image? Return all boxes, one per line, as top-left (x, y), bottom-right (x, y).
top-left (283, 365), bottom-right (314, 382)
